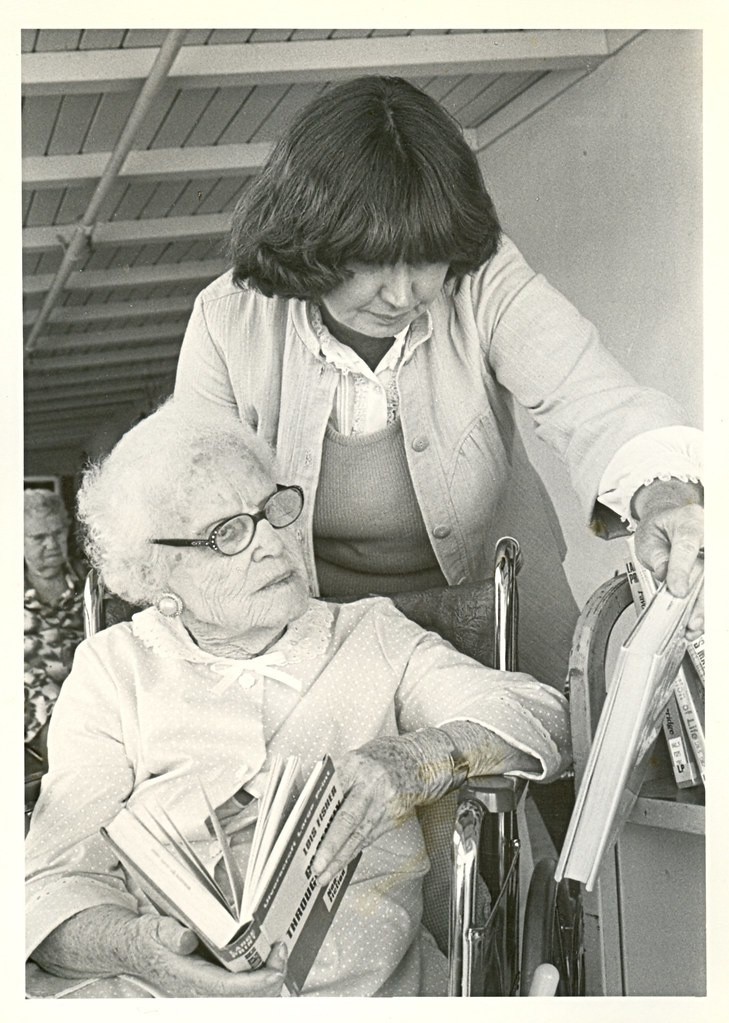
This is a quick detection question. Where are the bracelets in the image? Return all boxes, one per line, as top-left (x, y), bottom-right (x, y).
top-left (422, 726), bottom-right (469, 795)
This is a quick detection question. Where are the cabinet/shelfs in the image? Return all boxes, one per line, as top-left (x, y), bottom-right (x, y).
top-left (571, 576), bottom-right (708, 996)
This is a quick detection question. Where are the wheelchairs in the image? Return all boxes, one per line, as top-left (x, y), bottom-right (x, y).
top-left (77, 535), bottom-right (589, 997)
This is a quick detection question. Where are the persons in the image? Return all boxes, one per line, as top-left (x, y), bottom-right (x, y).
top-left (24, 489), bottom-right (90, 835)
top-left (23, 400), bottom-right (571, 997)
top-left (175, 76), bottom-right (703, 962)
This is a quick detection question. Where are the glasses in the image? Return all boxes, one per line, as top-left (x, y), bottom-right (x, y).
top-left (147, 483), bottom-right (305, 556)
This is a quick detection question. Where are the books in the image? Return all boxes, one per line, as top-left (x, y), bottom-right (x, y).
top-left (99, 755), bottom-right (362, 997)
top-left (555, 533), bottom-right (704, 891)
